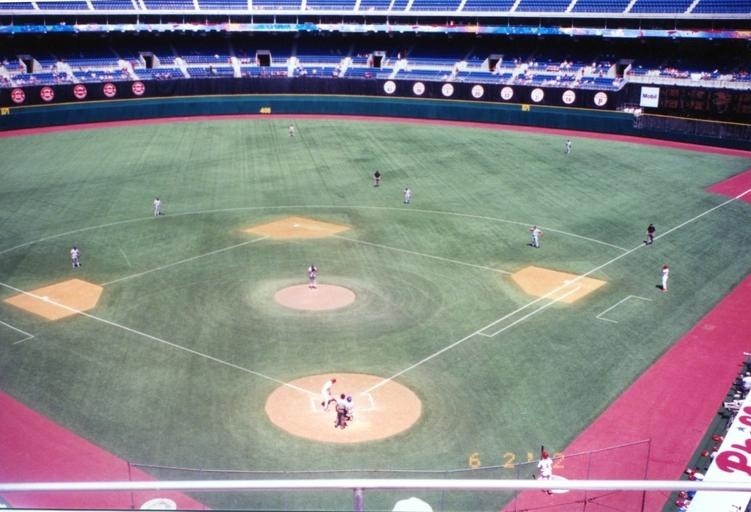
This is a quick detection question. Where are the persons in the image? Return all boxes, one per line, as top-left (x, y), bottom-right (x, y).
top-left (319, 377), bottom-right (335, 410)
top-left (645, 223), bottom-right (656, 246)
top-left (659, 263), bottom-right (670, 293)
top-left (68, 244), bottom-right (81, 269)
top-left (333, 393), bottom-right (349, 428)
top-left (152, 194), bottom-right (161, 217)
top-left (372, 169), bottom-right (381, 186)
top-left (403, 186), bottom-right (411, 204)
top-left (344, 395), bottom-right (356, 422)
top-left (527, 224), bottom-right (544, 249)
top-left (534, 450), bottom-right (553, 496)
top-left (563, 139), bottom-right (572, 155)
top-left (287, 122), bottom-right (295, 136)
top-left (672, 359), bottom-right (751, 512)
top-left (306, 263), bottom-right (318, 291)
